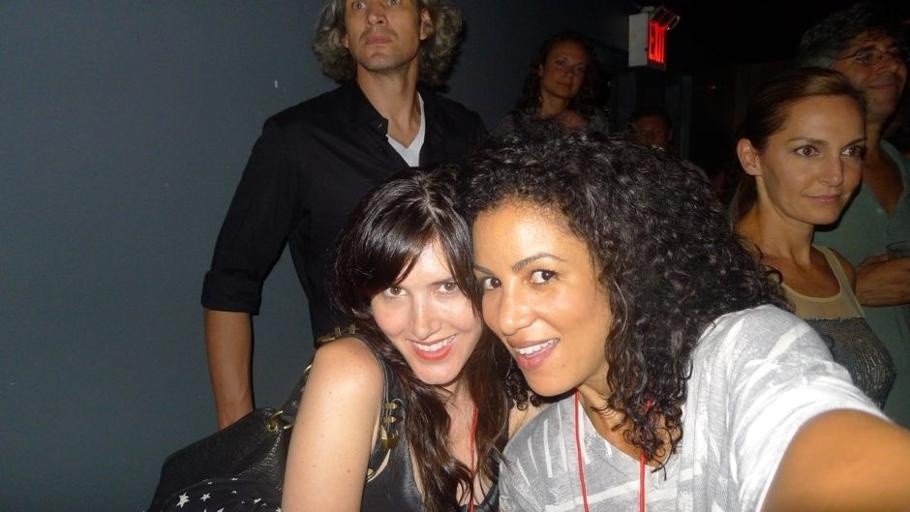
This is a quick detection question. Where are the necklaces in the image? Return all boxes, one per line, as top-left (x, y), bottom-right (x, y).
top-left (468, 405), bottom-right (478, 512)
top-left (572, 389), bottom-right (661, 511)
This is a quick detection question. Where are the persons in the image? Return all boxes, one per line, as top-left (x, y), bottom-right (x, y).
top-left (459, 112), bottom-right (910, 512)
top-left (276, 168), bottom-right (555, 511)
top-left (795, 6), bottom-right (910, 430)
top-left (487, 31), bottom-right (610, 156)
top-left (629, 106), bottom-right (671, 158)
top-left (201, 0), bottom-right (488, 432)
top-left (725, 67), bottom-right (896, 415)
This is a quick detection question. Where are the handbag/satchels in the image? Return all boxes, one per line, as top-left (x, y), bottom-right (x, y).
top-left (144, 322), bottom-right (409, 510)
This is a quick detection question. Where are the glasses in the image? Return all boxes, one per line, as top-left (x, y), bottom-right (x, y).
top-left (851, 46), bottom-right (904, 64)
